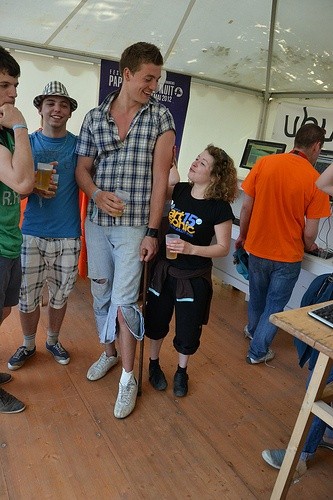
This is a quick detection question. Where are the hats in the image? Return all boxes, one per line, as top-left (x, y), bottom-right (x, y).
top-left (233, 247), bottom-right (250, 280)
top-left (33, 81), bottom-right (78, 112)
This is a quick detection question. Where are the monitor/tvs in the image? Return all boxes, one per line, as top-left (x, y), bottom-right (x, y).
top-left (239, 139), bottom-right (286, 169)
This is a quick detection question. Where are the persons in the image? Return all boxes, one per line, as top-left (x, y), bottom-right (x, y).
top-left (8, 80), bottom-right (78, 371)
top-left (74, 41), bottom-right (176, 419)
top-left (0, 45), bottom-right (57, 415)
top-left (235, 123), bottom-right (331, 368)
top-left (315, 163), bottom-right (333, 196)
top-left (142, 143), bottom-right (242, 398)
top-left (261, 367), bottom-right (333, 470)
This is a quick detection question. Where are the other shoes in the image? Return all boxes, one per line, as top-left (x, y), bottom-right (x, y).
top-left (261, 448), bottom-right (298, 470)
top-left (319, 438), bottom-right (333, 450)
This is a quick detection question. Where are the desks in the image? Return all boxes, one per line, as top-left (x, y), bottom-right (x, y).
top-left (212, 224), bottom-right (333, 312)
top-left (269, 301), bottom-right (333, 500)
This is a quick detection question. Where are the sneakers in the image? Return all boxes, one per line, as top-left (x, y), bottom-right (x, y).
top-left (113, 376), bottom-right (139, 419)
top-left (87, 349), bottom-right (118, 381)
top-left (173, 372), bottom-right (189, 396)
top-left (0, 372), bottom-right (12, 384)
top-left (246, 348), bottom-right (275, 368)
top-left (149, 365), bottom-right (167, 390)
top-left (0, 388), bottom-right (25, 412)
top-left (8, 344), bottom-right (37, 370)
top-left (46, 340), bottom-right (70, 365)
top-left (244, 324), bottom-right (254, 340)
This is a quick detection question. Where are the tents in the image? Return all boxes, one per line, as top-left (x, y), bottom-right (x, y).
top-left (0, 0), bottom-right (333, 183)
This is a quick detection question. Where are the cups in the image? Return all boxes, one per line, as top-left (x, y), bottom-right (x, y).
top-left (111, 190), bottom-right (129, 216)
top-left (45, 174), bottom-right (59, 197)
top-left (35, 162), bottom-right (53, 191)
top-left (166, 234), bottom-right (180, 259)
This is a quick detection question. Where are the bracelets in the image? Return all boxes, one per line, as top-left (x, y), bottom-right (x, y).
top-left (92, 189), bottom-right (102, 199)
top-left (12, 124), bottom-right (28, 130)
top-left (145, 228), bottom-right (159, 238)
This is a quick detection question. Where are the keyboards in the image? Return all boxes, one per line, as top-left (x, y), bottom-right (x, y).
top-left (308, 303), bottom-right (333, 329)
